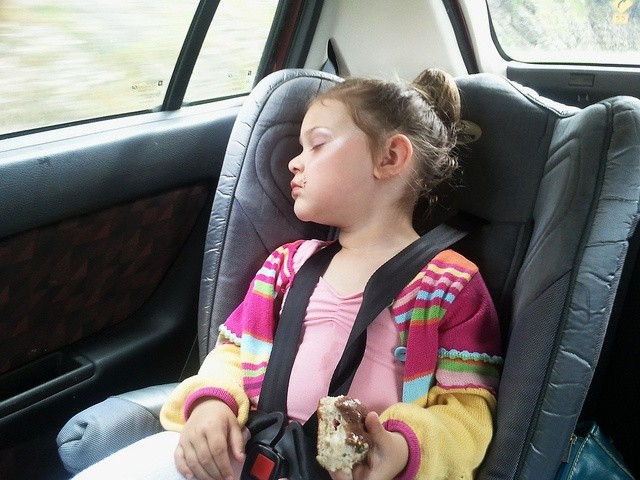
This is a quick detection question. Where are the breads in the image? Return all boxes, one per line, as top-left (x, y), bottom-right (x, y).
top-left (314, 394), bottom-right (376, 473)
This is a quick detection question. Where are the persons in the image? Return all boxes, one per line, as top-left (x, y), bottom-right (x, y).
top-left (158, 66), bottom-right (504, 480)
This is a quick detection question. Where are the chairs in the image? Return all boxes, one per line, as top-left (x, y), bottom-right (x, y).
top-left (56, 68), bottom-right (640, 480)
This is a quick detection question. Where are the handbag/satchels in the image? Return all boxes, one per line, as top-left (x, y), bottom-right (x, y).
top-left (559, 420), bottom-right (636, 480)
